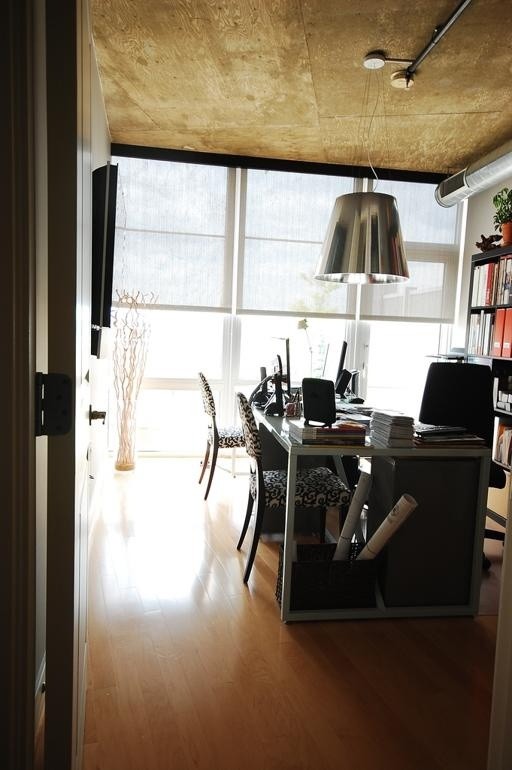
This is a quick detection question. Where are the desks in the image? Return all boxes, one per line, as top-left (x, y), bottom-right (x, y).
top-left (249, 397), bottom-right (492, 623)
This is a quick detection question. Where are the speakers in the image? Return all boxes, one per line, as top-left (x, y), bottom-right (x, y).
top-left (264, 377), bottom-right (285, 417)
top-left (254, 367), bottom-right (268, 402)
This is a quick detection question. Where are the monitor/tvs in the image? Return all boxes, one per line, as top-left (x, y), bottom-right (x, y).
top-left (248, 336), bottom-right (291, 412)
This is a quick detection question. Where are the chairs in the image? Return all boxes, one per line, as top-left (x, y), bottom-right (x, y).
top-left (234, 392), bottom-right (352, 584)
top-left (419, 362), bottom-right (507, 571)
top-left (196, 370), bottom-right (246, 500)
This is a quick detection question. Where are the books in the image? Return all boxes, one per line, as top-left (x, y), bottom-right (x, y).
top-left (468, 255), bottom-right (512, 358)
top-left (369, 410), bottom-right (485, 449)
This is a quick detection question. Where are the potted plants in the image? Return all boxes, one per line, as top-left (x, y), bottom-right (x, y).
top-left (492, 187), bottom-right (512, 245)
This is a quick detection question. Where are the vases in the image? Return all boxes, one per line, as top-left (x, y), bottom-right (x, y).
top-left (114, 398), bottom-right (140, 471)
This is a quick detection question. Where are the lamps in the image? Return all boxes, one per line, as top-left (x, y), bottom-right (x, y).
top-left (312, 56), bottom-right (410, 286)
top-left (296, 319), bottom-right (313, 378)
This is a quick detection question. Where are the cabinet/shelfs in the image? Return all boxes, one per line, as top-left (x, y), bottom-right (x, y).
top-left (463, 245), bottom-right (512, 528)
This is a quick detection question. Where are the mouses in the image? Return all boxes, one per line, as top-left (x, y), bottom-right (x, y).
top-left (349, 397), bottom-right (364, 404)
